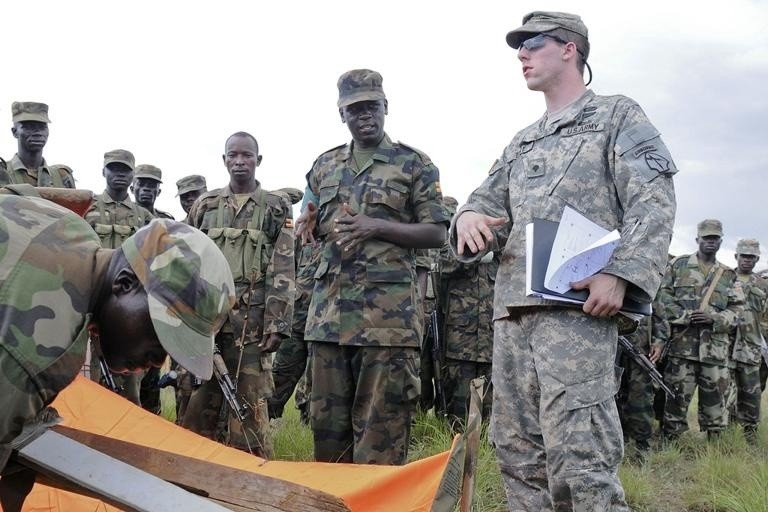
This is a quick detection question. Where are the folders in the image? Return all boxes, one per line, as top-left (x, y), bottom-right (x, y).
top-left (524, 218), bottom-right (651, 323)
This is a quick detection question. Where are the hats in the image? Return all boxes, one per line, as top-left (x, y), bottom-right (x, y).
top-left (120, 216), bottom-right (238, 383)
top-left (735, 236), bottom-right (762, 260)
top-left (132, 163), bottom-right (164, 185)
top-left (172, 173), bottom-right (209, 201)
top-left (694, 216), bottom-right (726, 242)
top-left (335, 67), bottom-right (387, 110)
top-left (102, 148), bottom-right (136, 172)
top-left (9, 99), bottom-right (55, 127)
top-left (504, 8), bottom-right (592, 66)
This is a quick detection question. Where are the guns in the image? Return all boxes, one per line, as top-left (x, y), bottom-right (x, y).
top-left (429, 272), bottom-right (447, 416)
top-left (191, 344), bottom-right (246, 421)
top-left (617, 335), bottom-right (678, 400)
top-left (91, 336), bottom-right (124, 394)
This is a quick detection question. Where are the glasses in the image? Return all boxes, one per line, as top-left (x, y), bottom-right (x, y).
top-left (519, 33), bottom-right (577, 52)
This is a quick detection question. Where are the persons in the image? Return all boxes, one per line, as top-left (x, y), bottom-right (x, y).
top-left (295, 69), bottom-right (496, 468)
top-left (0, 101), bottom-right (311, 512)
top-left (610, 219), bottom-right (768, 467)
top-left (447, 11), bottom-right (679, 511)
